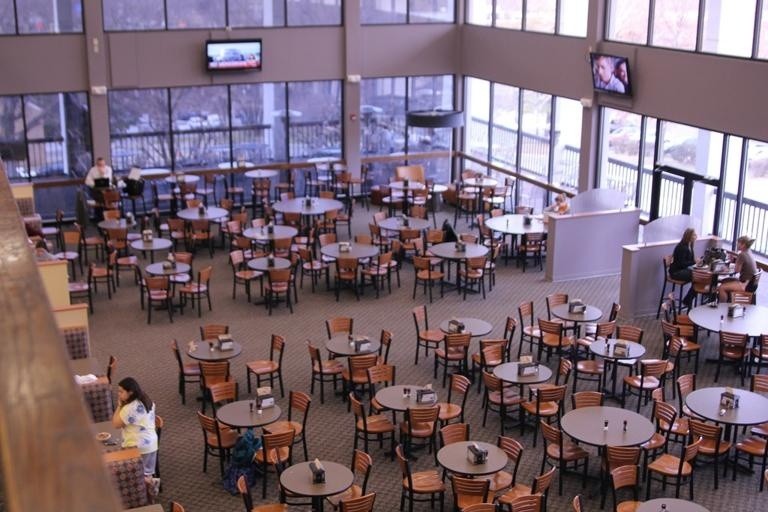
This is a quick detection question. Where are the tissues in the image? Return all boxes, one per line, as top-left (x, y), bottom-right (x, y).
top-left (419, 384), bottom-right (436, 406)
top-left (468, 443), bottom-right (487, 464)
top-left (722, 387), bottom-right (738, 411)
top-left (569, 298), bottom-right (585, 313)
top-left (257, 386), bottom-right (275, 409)
top-left (353, 335), bottom-right (370, 351)
top-left (309, 460), bottom-right (327, 485)
top-left (449, 316), bottom-right (465, 333)
top-left (518, 354), bottom-right (540, 377)
top-left (728, 300), bottom-right (744, 318)
top-left (614, 339), bottom-right (629, 357)
top-left (218, 334), bottom-right (232, 351)
top-left (129, 172), bottom-right (532, 271)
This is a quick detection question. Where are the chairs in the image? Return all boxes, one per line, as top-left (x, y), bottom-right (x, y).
top-left (254, 429), bottom-right (296, 499)
top-left (326, 253), bottom-right (768, 510)
top-left (210, 380), bottom-right (240, 410)
top-left (305, 340), bottom-right (348, 405)
top-left (197, 410), bottom-right (241, 476)
top-left (171, 341), bottom-right (203, 405)
top-left (238, 476), bottom-right (284, 512)
top-left (46, 308), bottom-right (186, 512)
top-left (8, 165), bottom-right (549, 316)
top-left (201, 324), bottom-right (228, 342)
top-left (245, 334), bottom-right (287, 399)
top-left (263, 390), bottom-right (308, 466)
top-left (199, 362), bottom-right (230, 413)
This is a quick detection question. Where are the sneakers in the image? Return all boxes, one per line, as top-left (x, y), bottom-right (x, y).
top-left (683, 297), bottom-right (693, 309)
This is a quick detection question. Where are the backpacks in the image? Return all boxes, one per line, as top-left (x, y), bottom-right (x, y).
top-left (232, 430), bottom-right (263, 467)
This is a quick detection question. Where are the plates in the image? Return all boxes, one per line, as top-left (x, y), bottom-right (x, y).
top-left (96, 432), bottom-right (111, 441)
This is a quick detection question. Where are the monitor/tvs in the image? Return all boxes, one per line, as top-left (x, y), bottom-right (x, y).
top-left (590, 53), bottom-right (631, 98)
top-left (206, 38), bottom-right (262, 71)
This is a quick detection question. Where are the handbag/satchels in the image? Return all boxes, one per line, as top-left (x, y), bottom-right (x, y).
top-left (703, 247), bottom-right (726, 264)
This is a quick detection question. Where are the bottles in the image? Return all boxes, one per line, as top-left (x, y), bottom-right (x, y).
top-left (481, 448), bottom-right (489, 462)
top-left (402, 387), bottom-right (411, 399)
top-left (209, 342), bottom-right (214, 352)
top-left (623, 420), bottom-right (627, 432)
top-left (258, 404), bottom-right (263, 415)
top-left (604, 419), bottom-right (609, 430)
top-left (719, 315), bottom-right (724, 324)
top-left (347, 335), bottom-right (354, 348)
top-left (660, 503), bottom-right (667, 512)
top-left (720, 408), bottom-right (726, 416)
top-left (249, 402), bottom-right (253, 411)
top-left (742, 306), bottom-right (746, 316)
top-left (604, 336), bottom-right (610, 354)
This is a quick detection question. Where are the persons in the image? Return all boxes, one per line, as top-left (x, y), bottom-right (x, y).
top-left (592, 54), bottom-right (629, 95)
top-left (544, 193), bottom-right (567, 250)
top-left (718, 236), bottom-right (758, 303)
top-left (85, 157), bottom-right (113, 236)
top-left (111, 377), bottom-right (161, 498)
top-left (668, 228), bottom-right (704, 308)
top-left (401, 219), bottom-right (415, 259)
top-left (704, 236), bottom-right (727, 302)
top-left (442, 218), bottom-right (458, 241)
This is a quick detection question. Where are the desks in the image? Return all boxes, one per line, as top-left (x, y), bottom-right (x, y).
top-left (217, 399), bottom-right (281, 437)
top-left (280, 459), bottom-right (354, 508)
top-left (186, 339), bottom-right (243, 400)
